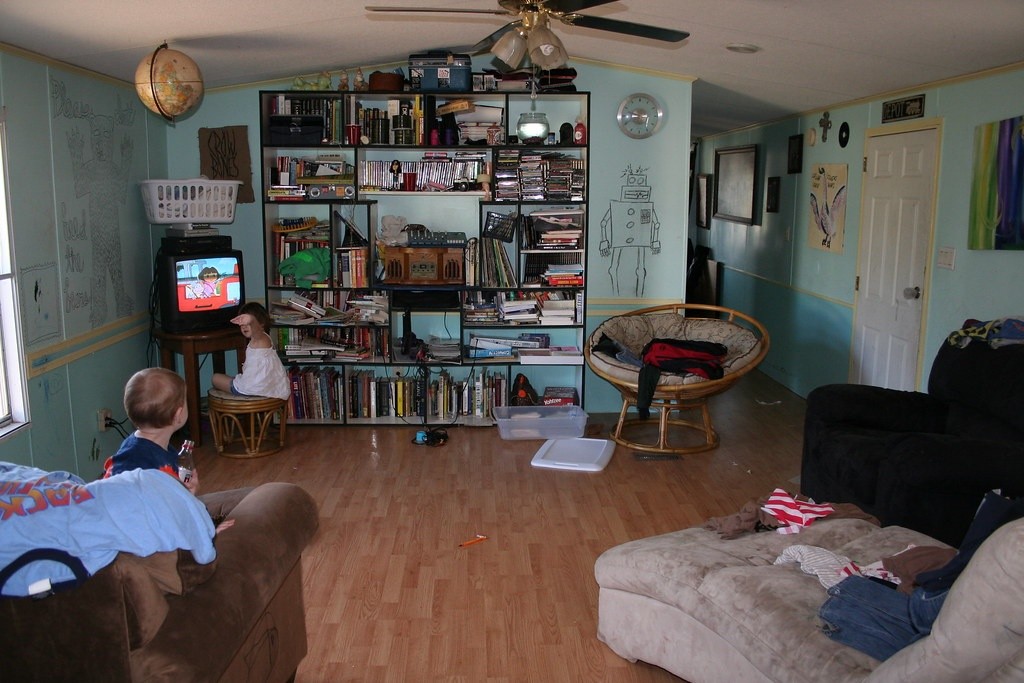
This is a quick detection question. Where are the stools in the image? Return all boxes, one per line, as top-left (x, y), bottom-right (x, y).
top-left (208, 388), bottom-right (286, 459)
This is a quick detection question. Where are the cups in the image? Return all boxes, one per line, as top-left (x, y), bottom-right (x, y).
top-left (404, 173), bottom-right (416, 191)
top-left (347, 125), bottom-right (361, 145)
top-left (488, 126), bottom-right (501, 145)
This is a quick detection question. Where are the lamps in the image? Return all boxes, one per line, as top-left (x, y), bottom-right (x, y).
top-left (490, 8), bottom-right (570, 71)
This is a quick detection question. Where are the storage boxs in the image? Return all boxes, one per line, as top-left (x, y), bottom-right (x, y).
top-left (464, 333), bottom-right (584, 364)
top-left (492, 407), bottom-right (588, 441)
top-left (543, 387), bottom-right (577, 406)
top-left (137, 178), bottom-right (244, 225)
top-left (529, 209), bottom-right (585, 231)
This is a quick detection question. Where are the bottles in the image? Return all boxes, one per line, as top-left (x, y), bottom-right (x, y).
top-left (575, 119), bottom-right (586, 145)
top-left (178, 440), bottom-right (195, 485)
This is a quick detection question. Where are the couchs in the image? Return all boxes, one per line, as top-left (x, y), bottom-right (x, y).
top-left (0, 462), bottom-right (319, 683)
top-left (593, 518), bottom-right (1024, 683)
top-left (801, 316), bottom-right (1024, 549)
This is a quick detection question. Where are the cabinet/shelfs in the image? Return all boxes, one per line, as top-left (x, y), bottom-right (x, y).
top-left (258, 91), bottom-right (591, 428)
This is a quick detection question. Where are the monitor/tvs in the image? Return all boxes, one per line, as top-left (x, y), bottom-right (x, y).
top-left (156, 249), bottom-right (246, 336)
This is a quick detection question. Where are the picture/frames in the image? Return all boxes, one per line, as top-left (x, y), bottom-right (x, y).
top-left (695, 174), bottom-right (712, 230)
top-left (471, 72), bottom-right (498, 91)
top-left (712, 144), bottom-right (759, 226)
top-left (766, 176), bottom-right (781, 213)
top-left (786, 133), bottom-right (804, 174)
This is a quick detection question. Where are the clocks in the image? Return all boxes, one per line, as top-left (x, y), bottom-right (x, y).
top-left (616, 93), bottom-right (664, 139)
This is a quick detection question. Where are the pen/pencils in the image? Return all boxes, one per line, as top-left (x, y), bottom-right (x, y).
top-left (458, 536), bottom-right (485, 547)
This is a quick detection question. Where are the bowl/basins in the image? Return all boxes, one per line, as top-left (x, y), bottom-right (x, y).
top-left (369, 73), bottom-right (404, 90)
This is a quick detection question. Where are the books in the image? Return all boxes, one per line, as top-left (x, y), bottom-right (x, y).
top-left (464, 289), bottom-right (582, 325)
top-left (333, 250), bottom-right (368, 288)
top-left (277, 289), bottom-right (389, 362)
top-left (347, 95), bottom-right (424, 145)
top-left (460, 126), bottom-right (504, 141)
top-left (268, 155), bottom-right (306, 201)
top-left (283, 365), bottom-right (507, 421)
top-left (272, 96), bottom-right (344, 144)
top-left (274, 233), bottom-right (328, 288)
top-left (466, 208), bottom-right (584, 287)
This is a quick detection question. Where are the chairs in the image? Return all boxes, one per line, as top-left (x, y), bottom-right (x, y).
top-left (584, 303), bottom-right (770, 454)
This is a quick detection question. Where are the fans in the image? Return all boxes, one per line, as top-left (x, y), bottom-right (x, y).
top-left (365, 0), bottom-right (691, 52)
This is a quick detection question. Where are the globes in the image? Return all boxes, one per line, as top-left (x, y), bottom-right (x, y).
top-left (135, 41), bottom-right (204, 119)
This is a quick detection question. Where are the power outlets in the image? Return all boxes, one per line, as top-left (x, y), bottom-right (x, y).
top-left (97, 408), bottom-right (112, 433)
top-left (392, 366), bottom-right (403, 377)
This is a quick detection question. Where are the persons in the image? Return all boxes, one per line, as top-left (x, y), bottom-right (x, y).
top-left (99, 368), bottom-right (235, 644)
top-left (212, 302), bottom-right (289, 397)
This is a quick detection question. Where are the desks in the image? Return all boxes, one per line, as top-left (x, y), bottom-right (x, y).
top-left (153, 326), bottom-right (250, 447)
